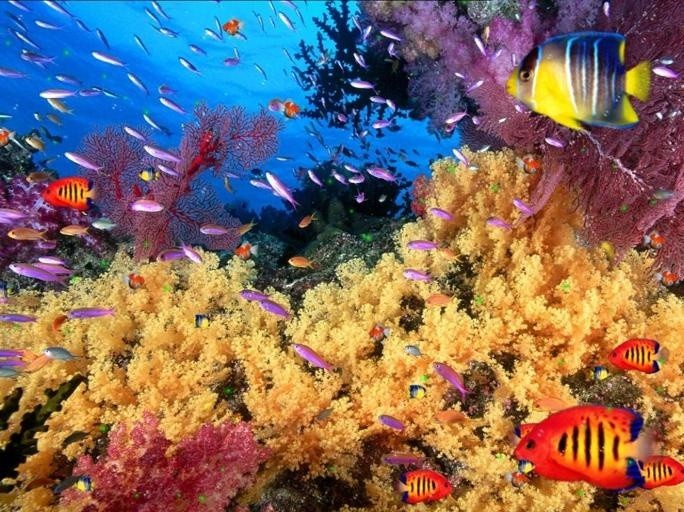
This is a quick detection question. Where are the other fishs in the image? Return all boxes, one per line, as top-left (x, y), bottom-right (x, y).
top-left (0, 0), bottom-right (682, 512)
